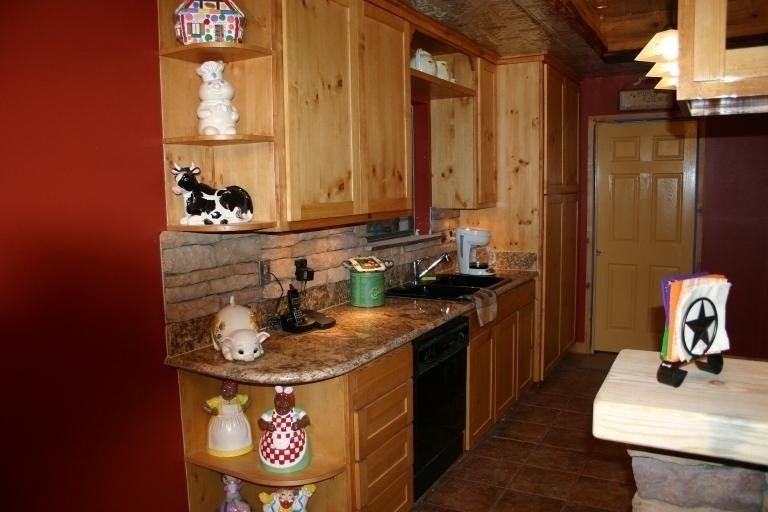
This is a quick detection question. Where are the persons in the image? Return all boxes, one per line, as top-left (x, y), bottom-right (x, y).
top-left (200, 381), bottom-right (253, 460)
top-left (255, 386), bottom-right (313, 474)
top-left (219, 474), bottom-right (251, 511)
top-left (257, 482), bottom-right (316, 512)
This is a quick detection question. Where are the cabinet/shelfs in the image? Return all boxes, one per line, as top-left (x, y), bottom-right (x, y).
top-left (459, 55), bottom-right (582, 387)
top-left (158, 0), bottom-right (498, 233)
top-left (176, 280), bottom-right (534, 512)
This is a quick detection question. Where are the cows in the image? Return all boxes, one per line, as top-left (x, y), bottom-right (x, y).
top-left (169, 161), bottom-right (254, 226)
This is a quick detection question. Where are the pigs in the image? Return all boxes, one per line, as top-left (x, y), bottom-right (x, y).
top-left (210, 295), bottom-right (271, 363)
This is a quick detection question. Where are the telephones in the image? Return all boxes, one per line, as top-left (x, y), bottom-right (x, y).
top-left (280, 284), bottom-right (316, 333)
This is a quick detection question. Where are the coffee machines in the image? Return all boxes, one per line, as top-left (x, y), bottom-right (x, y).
top-left (455, 226), bottom-right (496, 276)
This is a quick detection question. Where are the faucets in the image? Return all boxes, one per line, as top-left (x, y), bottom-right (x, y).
top-left (409, 253), bottom-right (450, 285)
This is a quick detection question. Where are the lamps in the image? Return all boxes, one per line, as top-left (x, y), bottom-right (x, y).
top-left (635, 29), bottom-right (679, 62)
top-left (646, 62), bottom-right (678, 77)
top-left (654, 77), bottom-right (677, 90)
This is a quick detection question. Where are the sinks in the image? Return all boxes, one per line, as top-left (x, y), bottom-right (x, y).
top-left (418, 273), bottom-right (514, 290)
top-left (383, 284), bottom-right (481, 303)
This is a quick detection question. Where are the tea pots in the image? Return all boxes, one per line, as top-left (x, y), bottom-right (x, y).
top-left (411, 48), bottom-right (438, 76)
